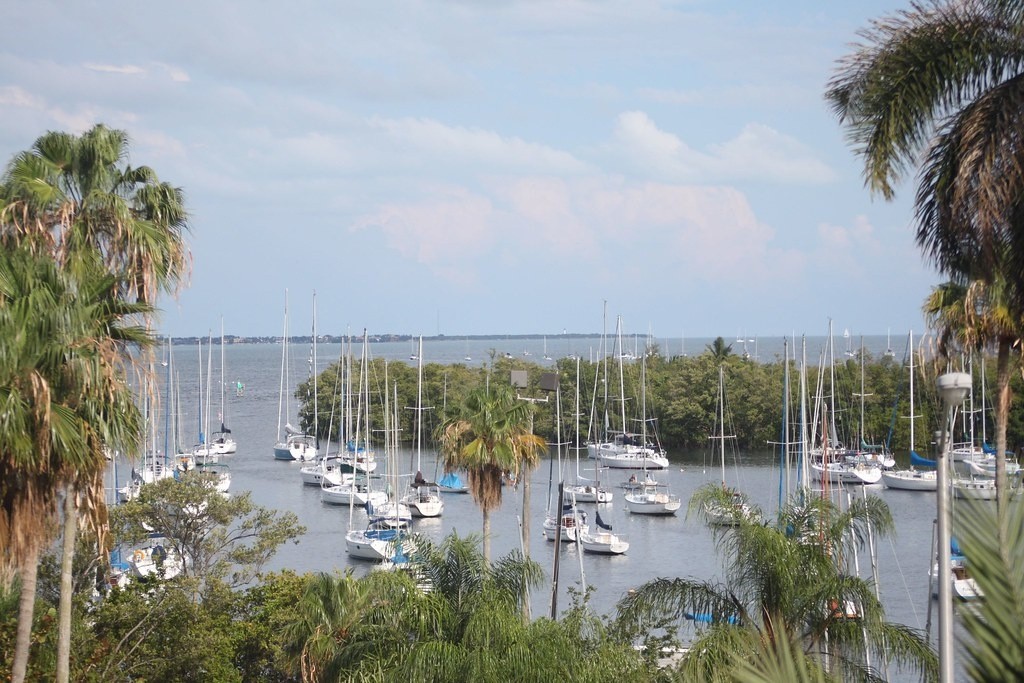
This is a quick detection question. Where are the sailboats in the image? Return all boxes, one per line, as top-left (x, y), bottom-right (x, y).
top-left (272, 287), bottom-right (472, 600)
top-left (543, 298), bottom-right (669, 543)
top-left (83, 314), bottom-right (237, 614)
top-left (930, 403), bottom-right (987, 602)
top-left (759, 316), bottom-right (1024, 635)
top-left (701, 365), bottom-right (755, 527)
top-left (580, 407), bottom-right (631, 555)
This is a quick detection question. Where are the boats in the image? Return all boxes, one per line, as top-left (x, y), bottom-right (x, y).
top-left (632, 608), bottom-right (741, 672)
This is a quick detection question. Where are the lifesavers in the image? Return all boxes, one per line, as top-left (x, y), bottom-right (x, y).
top-left (598, 493), bottom-right (606, 502)
top-left (654, 453), bottom-right (659, 460)
top-left (134, 549), bottom-right (145, 560)
